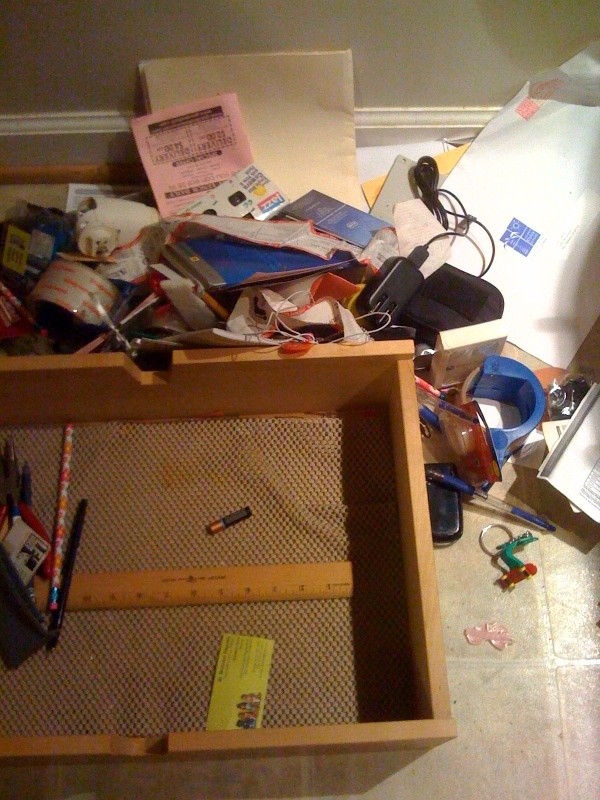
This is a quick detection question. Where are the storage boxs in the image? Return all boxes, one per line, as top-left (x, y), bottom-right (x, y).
top-left (429, 319), bottom-right (509, 391)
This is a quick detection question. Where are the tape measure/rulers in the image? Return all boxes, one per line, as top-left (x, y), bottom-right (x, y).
top-left (34, 559), bottom-right (355, 612)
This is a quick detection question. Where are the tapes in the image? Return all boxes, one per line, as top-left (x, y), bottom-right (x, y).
top-left (461, 362), bottom-right (491, 432)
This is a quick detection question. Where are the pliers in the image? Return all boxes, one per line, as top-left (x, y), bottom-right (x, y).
top-left (1, 442), bottom-right (54, 581)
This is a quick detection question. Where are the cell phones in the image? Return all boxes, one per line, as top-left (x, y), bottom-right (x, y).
top-left (424, 461), bottom-right (462, 541)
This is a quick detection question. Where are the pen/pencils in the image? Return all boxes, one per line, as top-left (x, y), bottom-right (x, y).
top-left (412, 375), bottom-right (447, 400)
top-left (417, 388), bottom-right (479, 424)
top-left (0, 542), bottom-right (43, 624)
top-left (7, 492), bottom-right (21, 526)
top-left (416, 403), bottom-right (444, 434)
top-left (49, 498), bottom-right (89, 649)
top-left (21, 460), bottom-right (33, 503)
top-left (48, 422), bottom-right (77, 608)
top-left (424, 469), bottom-right (557, 535)
